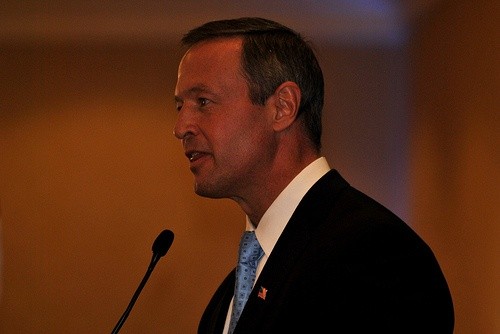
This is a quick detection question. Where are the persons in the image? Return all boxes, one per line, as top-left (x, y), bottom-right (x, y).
top-left (173, 17), bottom-right (454, 333)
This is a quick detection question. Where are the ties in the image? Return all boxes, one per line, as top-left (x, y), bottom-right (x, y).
top-left (227, 230), bottom-right (264, 334)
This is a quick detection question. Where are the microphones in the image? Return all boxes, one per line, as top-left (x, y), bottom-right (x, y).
top-left (110, 229), bottom-right (175, 333)
